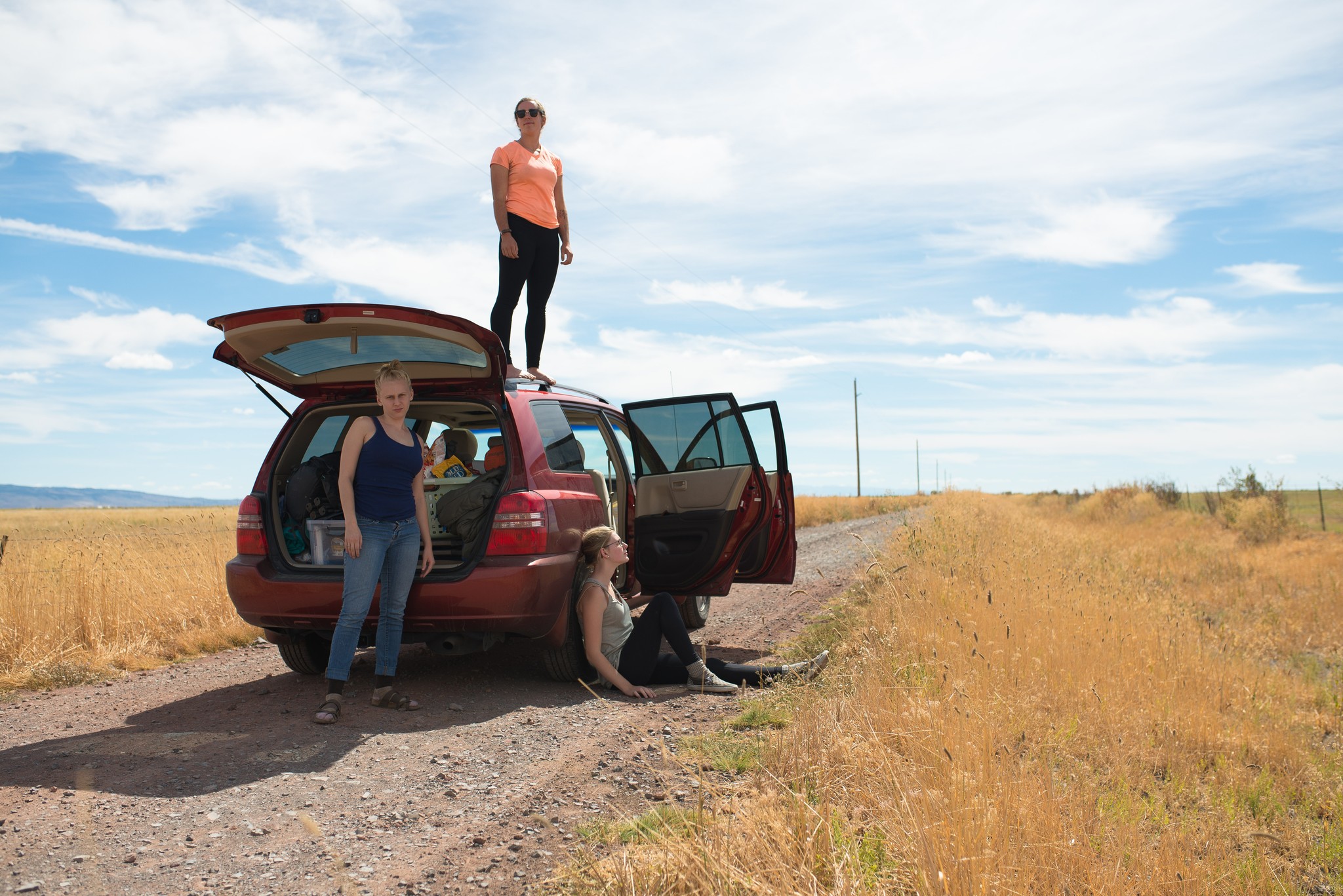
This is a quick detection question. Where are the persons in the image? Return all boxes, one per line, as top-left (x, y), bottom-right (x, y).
top-left (575, 526), bottom-right (830, 698)
top-left (489, 96), bottom-right (573, 386)
top-left (313, 360), bottom-right (435, 724)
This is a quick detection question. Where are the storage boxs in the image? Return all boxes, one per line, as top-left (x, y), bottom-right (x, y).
top-left (423, 476), bottom-right (483, 538)
top-left (306, 520), bottom-right (346, 564)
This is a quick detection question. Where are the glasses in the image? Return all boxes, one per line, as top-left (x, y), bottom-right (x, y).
top-left (516, 109), bottom-right (544, 119)
top-left (601, 539), bottom-right (625, 559)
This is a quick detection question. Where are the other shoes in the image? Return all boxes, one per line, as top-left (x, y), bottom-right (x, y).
top-left (687, 671), bottom-right (738, 692)
top-left (803, 651), bottom-right (830, 682)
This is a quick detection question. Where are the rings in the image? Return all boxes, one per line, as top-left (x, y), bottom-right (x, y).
top-left (347, 544), bottom-right (351, 546)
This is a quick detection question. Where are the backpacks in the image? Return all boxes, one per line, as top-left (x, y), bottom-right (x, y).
top-left (285, 451), bottom-right (340, 521)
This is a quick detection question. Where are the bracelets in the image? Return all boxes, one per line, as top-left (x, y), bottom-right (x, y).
top-left (500, 229), bottom-right (511, 236)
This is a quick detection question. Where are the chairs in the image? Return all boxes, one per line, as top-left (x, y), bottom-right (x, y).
top-left (547, 438), bottom-right (615, 526)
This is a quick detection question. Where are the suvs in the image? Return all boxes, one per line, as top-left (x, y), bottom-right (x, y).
top-left (205, 300), bottom-right (800, 673)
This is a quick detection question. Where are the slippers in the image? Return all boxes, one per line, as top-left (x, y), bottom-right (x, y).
top-left (313, 698), bottom-right (342, 724)
top-left (373, 690), bottom-right (420, 712)
